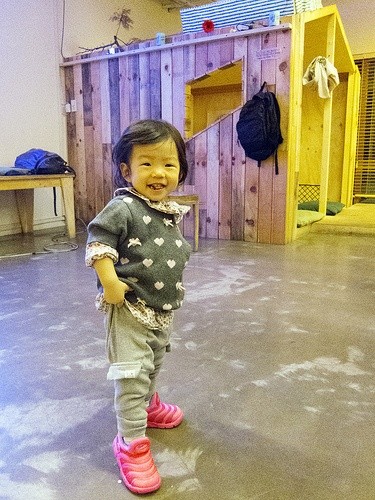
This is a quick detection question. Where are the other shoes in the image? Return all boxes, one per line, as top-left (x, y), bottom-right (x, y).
top-left (145, 392), bottom-right (184, 428)
top-left (113, 434), bottom-right (162, 494)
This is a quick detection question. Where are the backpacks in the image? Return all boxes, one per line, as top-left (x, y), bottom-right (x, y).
top-left (235, 80), bottom-right (284, 175)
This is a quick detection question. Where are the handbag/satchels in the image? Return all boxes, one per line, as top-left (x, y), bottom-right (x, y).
top-left (14, 147), bottom-right (77, 180)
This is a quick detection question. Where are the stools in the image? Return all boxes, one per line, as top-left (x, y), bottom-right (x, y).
top-left (168, 192), bottom-right (199, 251)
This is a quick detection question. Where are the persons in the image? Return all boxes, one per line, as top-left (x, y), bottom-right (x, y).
top-left (85, 118), bottom-right (192, 494)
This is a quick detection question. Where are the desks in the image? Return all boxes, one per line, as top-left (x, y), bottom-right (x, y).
top-left (0, 174), bottom-right (76, 239)
